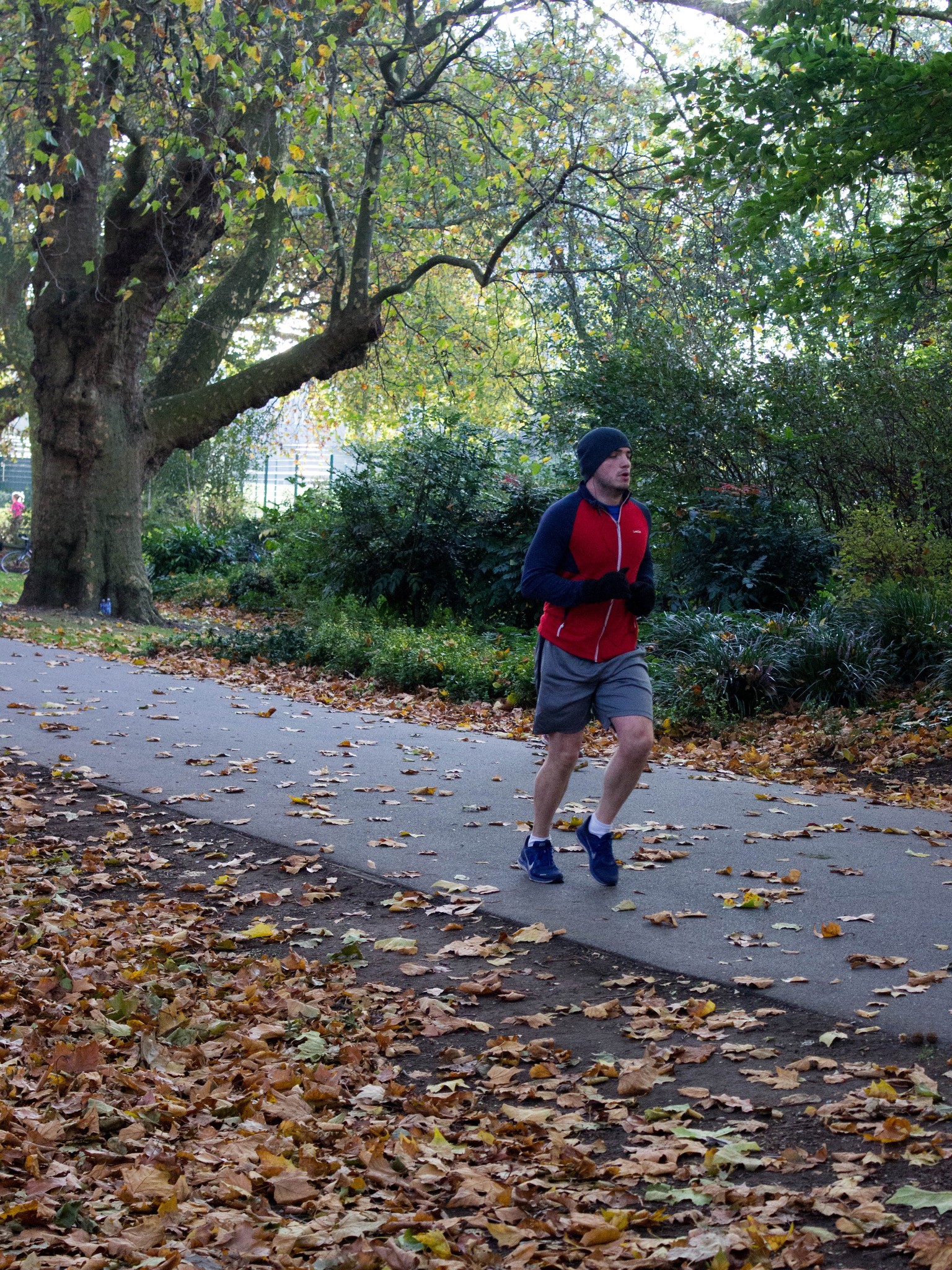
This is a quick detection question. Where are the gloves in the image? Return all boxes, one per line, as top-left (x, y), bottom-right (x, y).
top-left (625, 581), bottom-right (655, 617)
top-left (583, 567), bottom-right (634, 604)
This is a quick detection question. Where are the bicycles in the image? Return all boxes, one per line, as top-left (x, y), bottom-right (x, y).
top-left (1, 533), bottom-right (33, 574)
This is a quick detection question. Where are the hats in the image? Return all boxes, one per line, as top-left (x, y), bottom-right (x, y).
top-left (576, 427), bottom-right (633, 484)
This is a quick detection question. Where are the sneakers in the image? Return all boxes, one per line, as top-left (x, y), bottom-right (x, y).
top-left (517, 835), bottom-right (564, 883)
top-left (576, 815), bottom-right (619, 887)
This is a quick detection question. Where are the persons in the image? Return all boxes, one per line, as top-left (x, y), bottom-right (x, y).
top-left (9, 491), bottom-right (25, 523)
top-left (517, 426), bottom-right (657, 887)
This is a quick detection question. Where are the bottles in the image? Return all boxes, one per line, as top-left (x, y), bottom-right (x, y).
top-left (105, 598), bottom-right (111, 616)
top-left (99, 600), bottom-right (105, 614)
